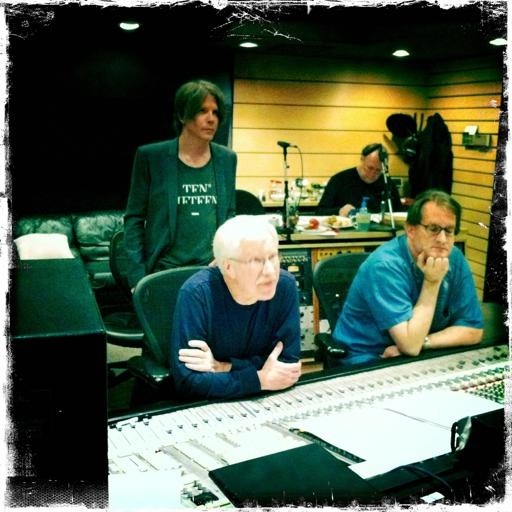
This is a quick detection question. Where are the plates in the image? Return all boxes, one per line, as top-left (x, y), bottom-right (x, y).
top-left (323, 219), bottom-right (353, 229)
top-left (301, 230), bottom-right (336, 237)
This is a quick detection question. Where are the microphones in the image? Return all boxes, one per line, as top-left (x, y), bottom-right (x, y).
top-left (277, 140), bottom-right (297, 148)
top-left (379, 148), bottom-right (384, 162)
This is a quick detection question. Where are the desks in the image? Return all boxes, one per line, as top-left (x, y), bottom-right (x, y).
top-left (276, 211), bottom-right (468, 250)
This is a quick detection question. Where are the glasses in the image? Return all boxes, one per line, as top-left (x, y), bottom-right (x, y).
top-left (228, 254), bottom-right (282, 271)
top-left (418, 222), bottom-right (456, 237)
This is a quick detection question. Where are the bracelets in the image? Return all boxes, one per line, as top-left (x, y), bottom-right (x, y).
top-left (422, 333), bottom-right (432, 349)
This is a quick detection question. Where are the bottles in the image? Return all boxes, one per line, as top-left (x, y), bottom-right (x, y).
top-left (268, 180), bottom-right (324, 204)
top-left (356, 196), bottom-right (372, 232)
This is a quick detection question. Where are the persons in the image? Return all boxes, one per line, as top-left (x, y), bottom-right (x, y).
top-left (331, 189), bottom-right (486, 368)
top-left (169, 210), bottom-right (305, 401)
top-left (314, 142), bottom-right (401, 223)
top-left (115, 75), bottom-right (238, 297)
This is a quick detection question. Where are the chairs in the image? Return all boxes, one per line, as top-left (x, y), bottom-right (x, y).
top-left (127, 264), bottom-right (213, 416)
top-left (312, 253), bottom-right (376, 375)
top-left (104, 230), bottom-right (147, 368)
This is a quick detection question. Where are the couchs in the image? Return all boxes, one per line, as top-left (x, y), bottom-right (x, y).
top-left (14, 207), bottom-right (127, 297)
